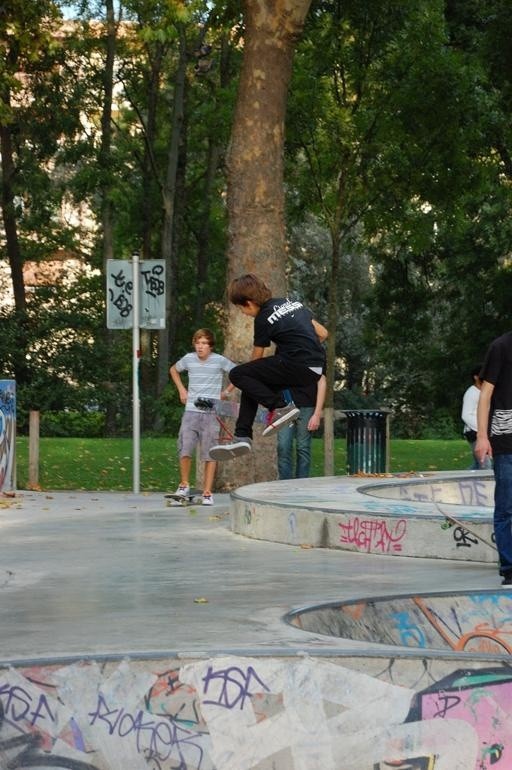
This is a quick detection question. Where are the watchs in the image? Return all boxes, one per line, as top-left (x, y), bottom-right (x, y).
top-left (224, 389), bottom-right (232, 397)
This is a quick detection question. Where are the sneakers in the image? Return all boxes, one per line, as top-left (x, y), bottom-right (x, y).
top-left (501, 573), bottom-right (512, 588)
top-left (263, 405), bottom-right (300, 437)
top-left (209, 438), bottom-right (252, 461)
top-left (202, 491), bottom-right (213, 505)
top-left (175, 481), bottom-right (190, 495)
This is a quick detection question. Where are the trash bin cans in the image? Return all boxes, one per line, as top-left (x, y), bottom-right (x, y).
top-left (343, 409), bottom-right (393, 474)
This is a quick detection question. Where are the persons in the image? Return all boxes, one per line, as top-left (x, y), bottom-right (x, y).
top-left (169, 329), bottom-right (237, 506)
top-left (474, 273), bottom-right (512, 585)
top-left (273, 347), bottom-right (327, 480)
top-left (461, 370), bottom-right (492, 469)
top-left (209, 274), bottom-right (328, 462)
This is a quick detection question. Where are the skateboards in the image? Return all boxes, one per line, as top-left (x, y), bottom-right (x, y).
top-left (432, 497), bottom-right (500, 570)
top-left (193, 396), bottom-right (273, 425)
top-left (164, 487), bottom-right (204, 506)
